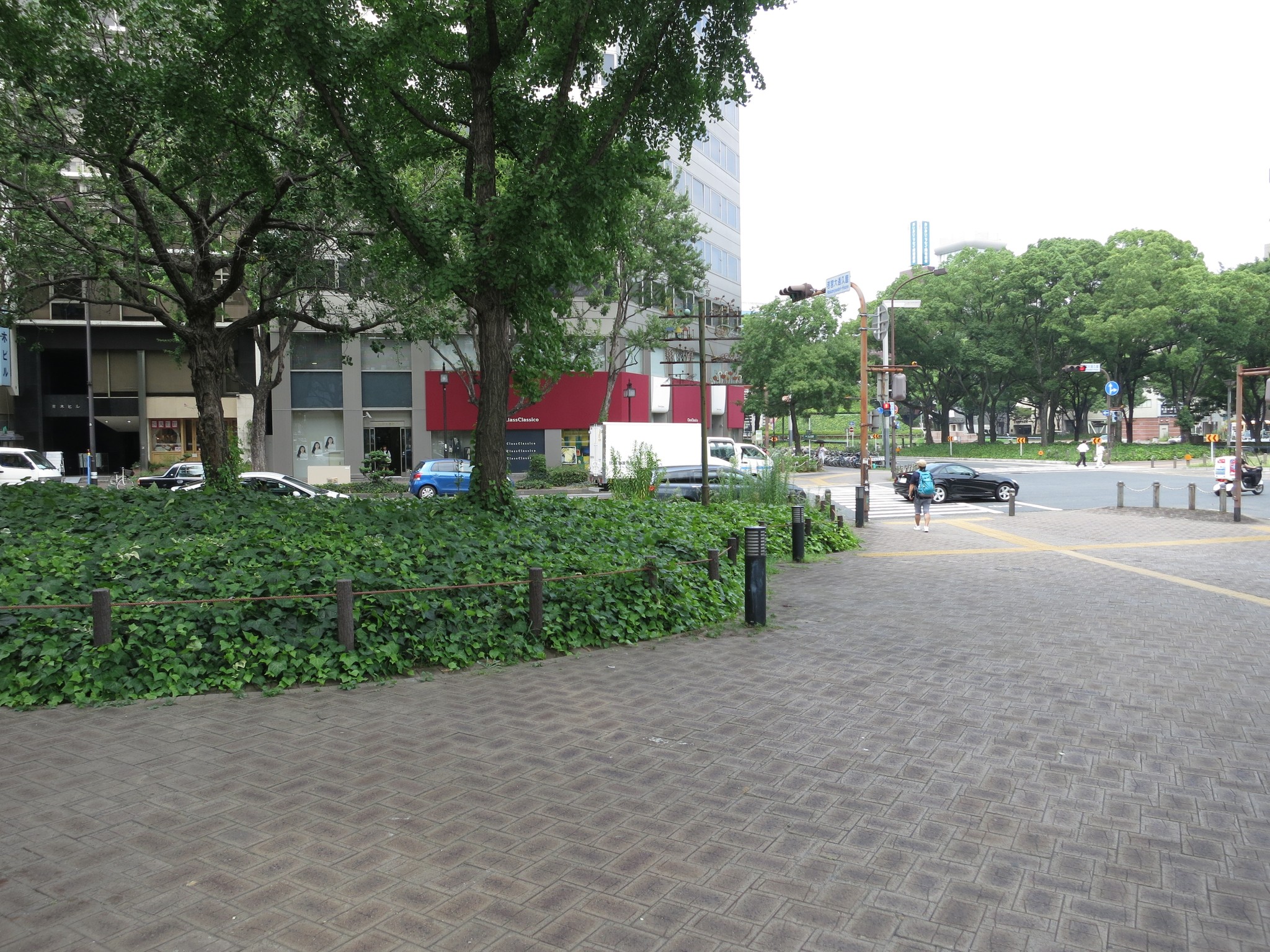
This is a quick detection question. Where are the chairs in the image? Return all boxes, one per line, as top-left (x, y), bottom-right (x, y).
top-left (255, 484), bottom-right (263, 491)
top-left (1241, 470), bottom-right (1252, 482)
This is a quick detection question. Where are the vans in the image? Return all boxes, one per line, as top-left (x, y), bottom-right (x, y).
top-left (1230, 420), bottom-right (1270, 442)
top-left (0, 446), bottom-right (62, 486)
top-left (710, 443), bottom-right (774, 480)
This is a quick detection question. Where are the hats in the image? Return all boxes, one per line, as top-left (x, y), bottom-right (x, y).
top-left (915, 459), bottom-right (927, 467)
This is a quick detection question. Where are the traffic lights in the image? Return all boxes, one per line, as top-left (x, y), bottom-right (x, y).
top-left (883, 403), bottom-right (891, 416)
top-left (778, 282), bottom-right (814, 303)
top-left (1063, 365), bottom-right (1086, 372)
top-left (1112, 411), bottom-right (1122, 422)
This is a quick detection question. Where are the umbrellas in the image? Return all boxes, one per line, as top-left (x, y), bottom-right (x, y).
top-left (814, 439), bottom-right (827, 449)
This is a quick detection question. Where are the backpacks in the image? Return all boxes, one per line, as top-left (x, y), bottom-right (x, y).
top-left (914, 470), bottom-right (936, 496)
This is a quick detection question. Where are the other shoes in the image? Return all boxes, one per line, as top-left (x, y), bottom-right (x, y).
top-left (923, 526), bottom-right (929, 532)
top-left (914, 525), bottom-right (921, 531)
top-left (1084, 465), bottom-right (1087, 467)
top-left (1093, 456), bottom-right (1097, 461)
top-left (1076, 464), bottom-right (1079, 467)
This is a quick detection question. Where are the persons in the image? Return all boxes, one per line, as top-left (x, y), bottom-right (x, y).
top-left (311, 442), bottom-right (323, 456)
top-left (816, 443), bottom-right (827, 467)
top-left (1075, 440), bottom-right (1089, 468)
top-left (908, 459), bottom-right (936, 532)
top-left (1240, 452), bottom-right (1258, 488)
top-left (1095, 442), bottom-right (1106, 468)
top-left (324, 436), bottom-right (336, 451)
top-left (297, 445), bottom-right (308, 460)
top-left (380, 445), bottom-right (391, 471)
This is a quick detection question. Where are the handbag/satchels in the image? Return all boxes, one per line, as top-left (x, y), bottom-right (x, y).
top-left (816, 447), bottom-right (820, 456)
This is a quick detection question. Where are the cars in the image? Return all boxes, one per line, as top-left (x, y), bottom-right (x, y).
top-left (171, 471), bottom-right (351, 500)
top-left (407, 458), bottom-right (515, 500)
top-left (137, 461), bottom-right (206, 490)
top-left (1167, 433), bottom-right (1200, 444)
top-left (649, 464), bottom-right (807, 504)
top-left (893, 461), bottom-right (1019, 504)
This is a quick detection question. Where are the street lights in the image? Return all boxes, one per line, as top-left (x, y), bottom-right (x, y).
top-left (890, 268), bottom-right (948, 472)
top-left (623, 378), bottom-right (636, 422)
top-left (1222, 379), bottom-right (1236, 446)
top-left (439, 362), bottom-right (449, 458)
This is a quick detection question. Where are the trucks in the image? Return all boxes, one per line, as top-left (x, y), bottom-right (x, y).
top-left (588, 422), bottom-right (740, 493)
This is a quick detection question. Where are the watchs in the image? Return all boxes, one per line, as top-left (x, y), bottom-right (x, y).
top-left (908, 495), bottom-right (911, 497)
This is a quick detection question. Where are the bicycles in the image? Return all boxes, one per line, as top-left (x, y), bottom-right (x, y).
top-left (108, 466), bottom-right (135, 491)
top-left (756, 444), bottom-right (881, 468)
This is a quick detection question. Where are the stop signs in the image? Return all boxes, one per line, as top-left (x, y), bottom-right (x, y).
top-left (849, 427), bottom-right (854, 432)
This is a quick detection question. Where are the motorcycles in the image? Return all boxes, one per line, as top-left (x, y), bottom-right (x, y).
top-left (1213, 446), bottom-right (1264, 497)
